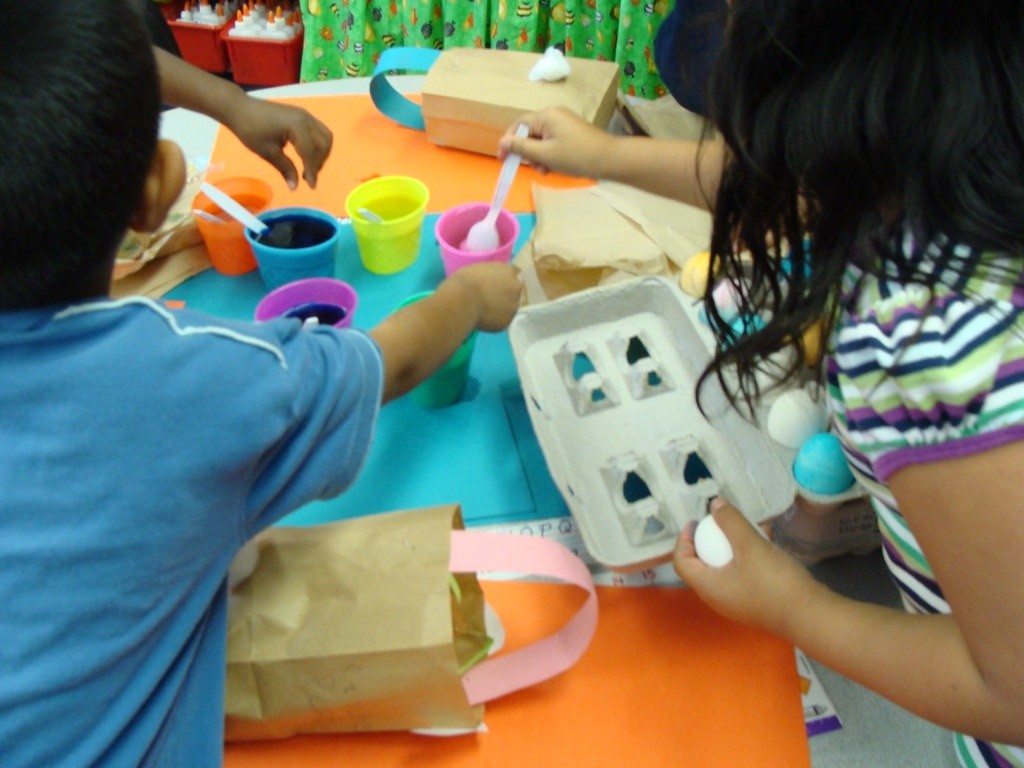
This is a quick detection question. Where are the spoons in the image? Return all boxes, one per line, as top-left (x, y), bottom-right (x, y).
top-left (466, 152), bottom-right (522, 251)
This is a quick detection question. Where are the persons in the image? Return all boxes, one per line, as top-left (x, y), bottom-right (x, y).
top-left (495, 0), bottom-right (1024, 768)
top-left (0, 0), bottom-right (524, 768)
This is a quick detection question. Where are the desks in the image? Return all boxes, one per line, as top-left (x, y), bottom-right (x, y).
top-left (161, 77), bottom-right (813, 767)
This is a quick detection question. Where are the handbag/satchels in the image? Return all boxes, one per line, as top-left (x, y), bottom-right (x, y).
top-left (222, 505), bottom-right (599, 747)
top-left (368, 45), bottom-right (621, 165)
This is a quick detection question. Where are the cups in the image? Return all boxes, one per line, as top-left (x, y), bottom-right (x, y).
top-left (391, 290), bottom-right (478, 408)
top-left (435, 201), bottom-right (519, 280)
top-left (255, 277), bottom-right (357, 333)
top-left (345, 175), bottom-right (430, 275)
top-left (192, 176), bottom-right (274, 276)
top-left (243, 206), bottom-right (340, 291)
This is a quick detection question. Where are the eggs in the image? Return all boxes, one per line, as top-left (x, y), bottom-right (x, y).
top-left (680, 251), bottom-right (854, 495)
top-left (695, 513), bottom-right (770, 567)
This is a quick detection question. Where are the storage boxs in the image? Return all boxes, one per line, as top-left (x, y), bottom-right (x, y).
top-left (160, 1), bottom-right (307, 86)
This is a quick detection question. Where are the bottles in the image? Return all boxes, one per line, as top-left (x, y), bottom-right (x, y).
top-left (177, 0), bottom-right (302, 41)
